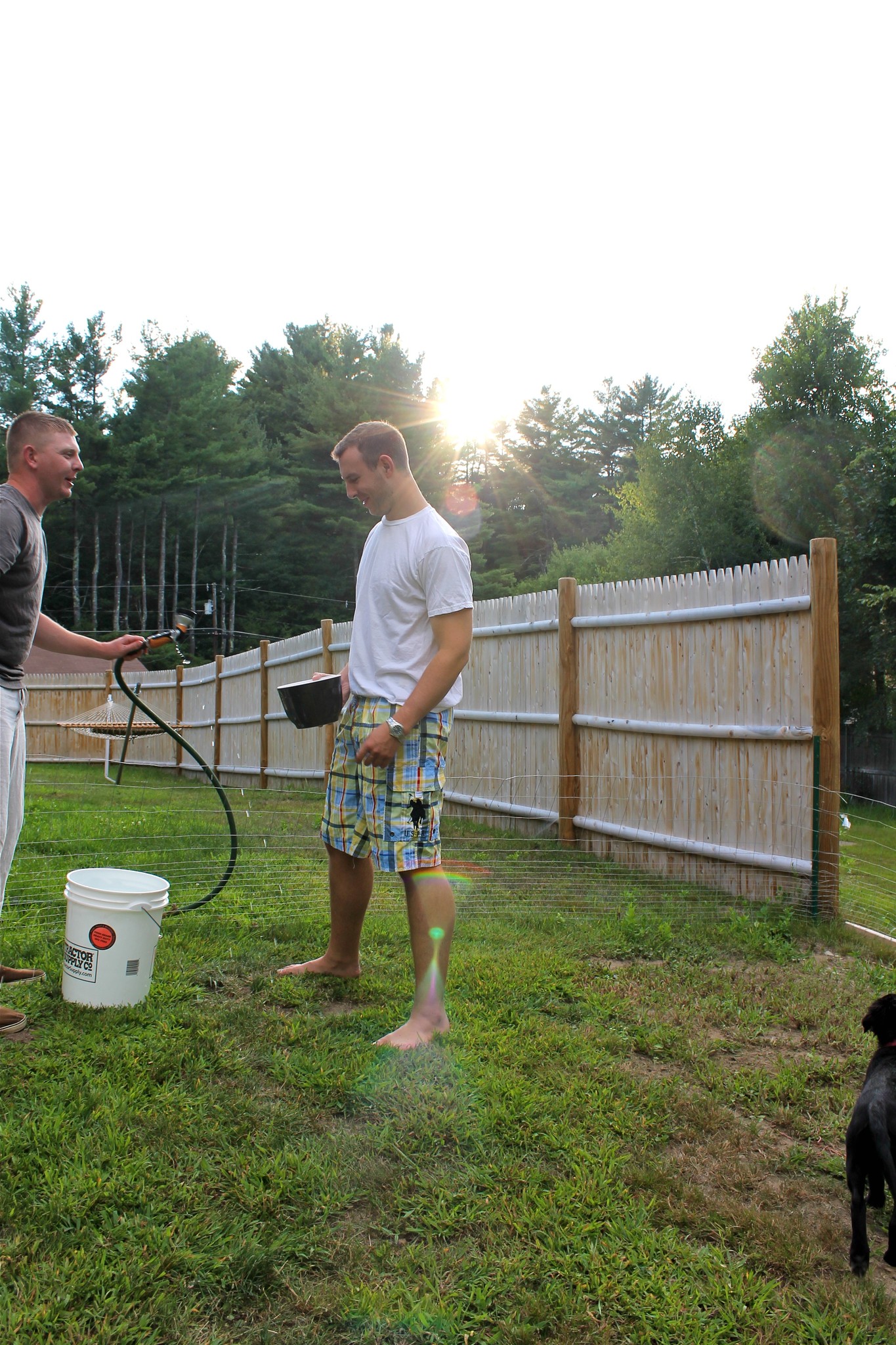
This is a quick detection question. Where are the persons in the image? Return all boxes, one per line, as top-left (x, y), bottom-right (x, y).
top-left (1, 413), bottom-right (149, 1031)
top-left (276, 419), bottom-right (472, 1050)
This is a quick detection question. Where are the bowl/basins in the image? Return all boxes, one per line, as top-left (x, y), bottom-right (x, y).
top-left (276, 674), bottom-right (342, 730)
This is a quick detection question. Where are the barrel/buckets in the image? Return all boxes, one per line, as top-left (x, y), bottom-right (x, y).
top-left (61, 866), bottom-right (170, 1010)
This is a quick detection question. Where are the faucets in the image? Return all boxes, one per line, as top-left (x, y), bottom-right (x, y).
top-left (143, 607), bottom-right (197, 665)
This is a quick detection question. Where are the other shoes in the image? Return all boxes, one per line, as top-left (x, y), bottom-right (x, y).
top-left (0, 964), bottom-right (45, 985)
top-left (0, 1005), bottom-right (27, 1034)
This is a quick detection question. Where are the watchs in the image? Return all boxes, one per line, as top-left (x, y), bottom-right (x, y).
top-left (386, 717), bottom-right (406, 744)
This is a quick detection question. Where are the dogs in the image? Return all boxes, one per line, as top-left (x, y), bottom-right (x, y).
top-left (846, 993), bottom-right (896, 1278)
